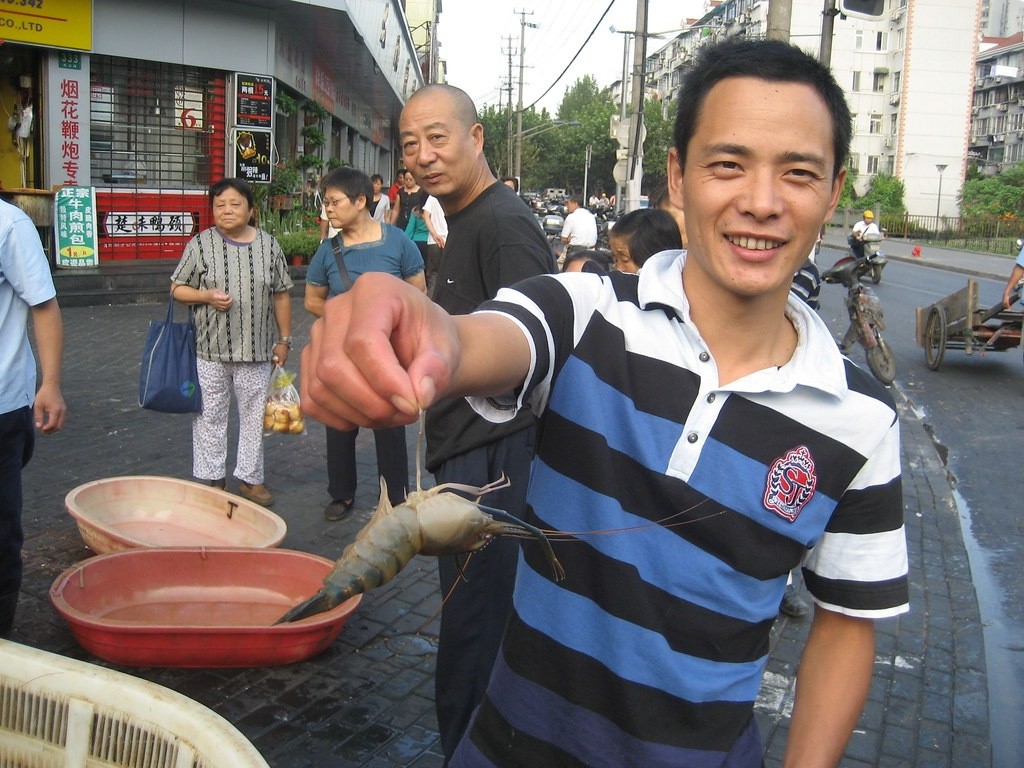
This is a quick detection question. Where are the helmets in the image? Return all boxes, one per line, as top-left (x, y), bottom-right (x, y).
top-left (863, 211), bottom-right (874, 219)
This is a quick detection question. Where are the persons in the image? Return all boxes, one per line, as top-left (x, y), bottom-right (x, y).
top-left (306, 84), bottom-right (829, 767)
top-left (852, 210), bottom-right (880, 269)
top-left (1002, 246), bottom-right (1024, 310)
top-left (169, 175), bottom-right (295, 505)
top-left (0, 199), bottom-right (67, 638)
top-left (301, 36), bottom-right (914, 768)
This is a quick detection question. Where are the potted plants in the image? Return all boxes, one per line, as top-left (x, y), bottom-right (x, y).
top-left (249, 92), bottom-right (353, 269)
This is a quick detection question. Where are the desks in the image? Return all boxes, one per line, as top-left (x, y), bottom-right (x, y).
top-left (0, 188), bottom-right (57, 269)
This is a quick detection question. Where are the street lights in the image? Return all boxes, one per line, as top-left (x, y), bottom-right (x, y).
top-left (508, 121), bottom-right (563, 178)
top-left (615, 34), bottom-right (666, 213)
top-left (514, 121), bottom-right (582, 197)
top-left (934, 163), bottom-right (948, 240)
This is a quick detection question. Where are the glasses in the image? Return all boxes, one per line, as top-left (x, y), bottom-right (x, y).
top-left (322, 196), bottom-right (347, 207)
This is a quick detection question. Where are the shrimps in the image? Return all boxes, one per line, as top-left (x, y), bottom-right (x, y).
top-left (270, 401), bottom-right (729, 635)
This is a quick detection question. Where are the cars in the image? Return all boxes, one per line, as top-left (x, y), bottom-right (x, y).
top-left (620, 196), bottom-right (649, 210)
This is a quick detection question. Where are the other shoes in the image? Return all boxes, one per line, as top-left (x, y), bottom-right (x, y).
top-left (240, 482), bottom-right (274, 506)
top-left (324, 499), bottom-right (354, 521)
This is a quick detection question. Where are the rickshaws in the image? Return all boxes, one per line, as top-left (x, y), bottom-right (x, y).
top-left (915, 279), bottom-right (1023, 370)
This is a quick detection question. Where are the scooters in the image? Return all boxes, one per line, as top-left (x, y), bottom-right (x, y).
top-left (847, 228), bottom-right (889, 284)
top-left (818, 250), bottom-right (896, 385)
top-left (537, 202), bottom-right (627, 272)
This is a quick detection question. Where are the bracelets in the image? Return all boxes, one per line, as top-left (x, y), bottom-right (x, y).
top-left (277, 336), bottom-right (294, 350)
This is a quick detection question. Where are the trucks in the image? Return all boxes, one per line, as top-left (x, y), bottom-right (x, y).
top-left (542, 187), bottom-right (566, 201)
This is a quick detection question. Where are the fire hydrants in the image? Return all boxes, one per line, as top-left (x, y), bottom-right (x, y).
top-left (912, 244), bottom-right (921, 257)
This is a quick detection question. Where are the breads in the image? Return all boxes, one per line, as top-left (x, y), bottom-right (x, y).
top-left (262, 403), bottom-right (304, 434)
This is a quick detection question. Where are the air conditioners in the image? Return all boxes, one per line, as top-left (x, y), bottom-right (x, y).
top-left (889, 12), bottom-right (900, 21)
top-left (976, 79), bottom-right (984, 87)
top-left (970, 136), bottom-right (977, 144)
top-left (889, 95), bottom-right (899, 105)
top-left (1018, 100), bottom-right (1024, 107)
top-left (1001, 104), bottom-right (1008, 112)
top-left (1016, 132), bottom-right (1023, 139)
top-left (884, 138), bottom-right (892, 147)
top-left (972, 106), bottom-right (978, 115)
top-left (997, 135), bottom-right (1005, 141)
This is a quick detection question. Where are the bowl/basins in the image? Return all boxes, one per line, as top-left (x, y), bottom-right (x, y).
top-left (49, 546), bottom-right (365, 668)
top-left (63, 474), bottom-right (287, 575)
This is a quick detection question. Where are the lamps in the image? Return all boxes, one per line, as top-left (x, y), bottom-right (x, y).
top-left (355, 30), bottom-right (364, 45)
top-left (372, 61), bottom-right (380, 75)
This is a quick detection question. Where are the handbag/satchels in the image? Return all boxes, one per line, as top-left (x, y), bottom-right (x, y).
top-left (138, 284), bottom-right (201, 413)
top-left (263, 364), bottom-right (307, 436)
